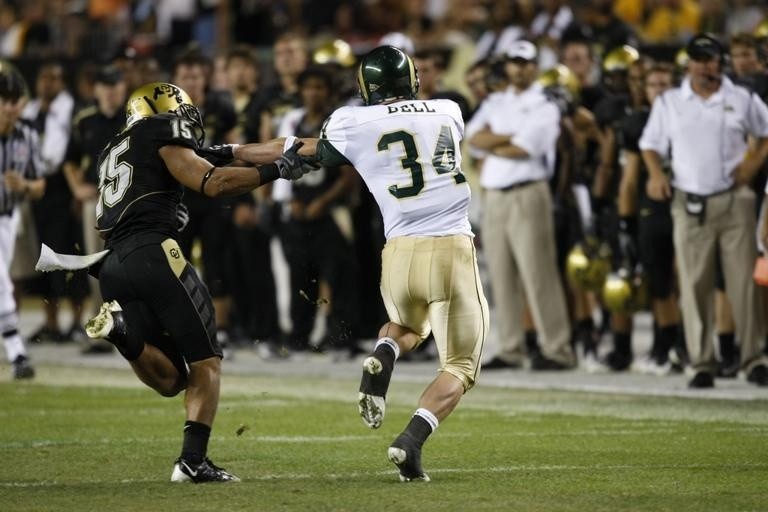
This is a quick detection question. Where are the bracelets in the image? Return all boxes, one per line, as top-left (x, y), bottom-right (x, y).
top-left (25, 181), bottom-right (29, 192)
top-left (284, 134), bottom-right (295, 154)
top-left (199, 166), bottom-right (214, 193)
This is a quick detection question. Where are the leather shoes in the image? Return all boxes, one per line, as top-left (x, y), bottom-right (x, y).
top-left (483, 340), bottom-right (768, 389)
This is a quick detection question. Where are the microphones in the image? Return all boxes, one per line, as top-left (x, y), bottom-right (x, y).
top-left (706, 75), bottom-right (723, 85)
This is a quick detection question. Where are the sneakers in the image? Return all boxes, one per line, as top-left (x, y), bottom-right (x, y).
top-left (85, 300), bottom-right (127, 341)
top-left (358, 354), bottom-right (391, 429)
top-left (388, 433), bottom-right (429, 482)
top-left (170, 456), bottom-right (240, 483)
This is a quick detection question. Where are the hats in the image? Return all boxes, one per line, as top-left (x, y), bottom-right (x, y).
top-left (688, 33), bottom-right (718, 56)
top-left (504, 39), bottom-right (538, 60)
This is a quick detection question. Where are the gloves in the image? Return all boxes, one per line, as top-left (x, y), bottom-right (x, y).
top-left (273, 141), bottom-right (321, 181)
top-left (198, 143), bottom-right (239, 167)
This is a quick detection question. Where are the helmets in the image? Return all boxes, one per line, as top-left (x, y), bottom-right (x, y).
top-left (310, 37), bottom-right (353, 68)
top-left (604, 41), bottom-right (637, 71)
top-left (567, 238), bottom-right (649, 311)
top-left (356, 46), bottom-right (420, 105)
top-left (125, 82), bottom-right (206, 147)
top-left (538, 65), bottom-right (579, 98)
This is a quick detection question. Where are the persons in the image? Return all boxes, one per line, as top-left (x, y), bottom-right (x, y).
top-left (638, 31), bottom-right (767, 389)
top-left (465, 40), bottom-right (577, 371)
top-left (2, 1), bottom-right (767, 378)
top-left (0, 62), bottom-right (45, 378)
top-left (202, 45), bottom-right (491, 482)
top-left (59, 74), bottom-right (131, 355)
top-left (85, 83), bottom-right (322, 483)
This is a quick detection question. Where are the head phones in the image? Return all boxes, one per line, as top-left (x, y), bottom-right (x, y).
top-left (687, 32), bottom-right (729, 72)
